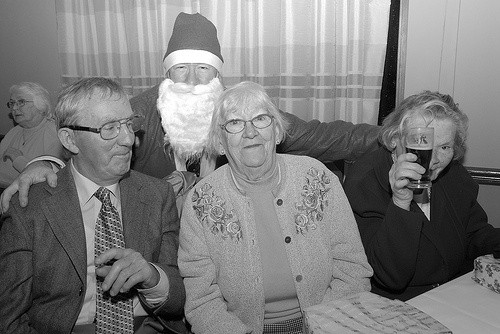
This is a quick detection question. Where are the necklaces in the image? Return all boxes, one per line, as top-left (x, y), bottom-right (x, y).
top-left (21, 120), bottom-right (47, 145)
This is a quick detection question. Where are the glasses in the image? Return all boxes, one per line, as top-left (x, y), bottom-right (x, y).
top-left (222, 114), bottom-right (274, 134)
top-left (60, 114), bottom-right (143, 140)
top-left (7, 98), bottom-right (33, 107)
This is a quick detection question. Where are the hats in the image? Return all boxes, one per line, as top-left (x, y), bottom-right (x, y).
top-left (162, 12), bottom-right (224, 75)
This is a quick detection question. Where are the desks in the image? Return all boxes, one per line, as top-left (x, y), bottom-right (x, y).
top-left (406, 259), bottom-right (500, 334)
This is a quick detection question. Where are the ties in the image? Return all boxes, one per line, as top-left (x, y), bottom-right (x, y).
top-left (94, 187), bottom-right (135, 333)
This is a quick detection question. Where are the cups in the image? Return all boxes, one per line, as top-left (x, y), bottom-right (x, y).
top-left (400, 127), bottom-right (434, 188)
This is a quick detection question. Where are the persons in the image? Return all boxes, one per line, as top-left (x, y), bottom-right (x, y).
top-left (177, 80), bottom-right (374, 334)
top-left (342, 90), bottom-right (500, 300)
top-left (0, 12), bottom-right (382, 219)
top-left (0, 82), bottom-right (75, 196)
top-left (0, 76), bottom-right (192, 334)
